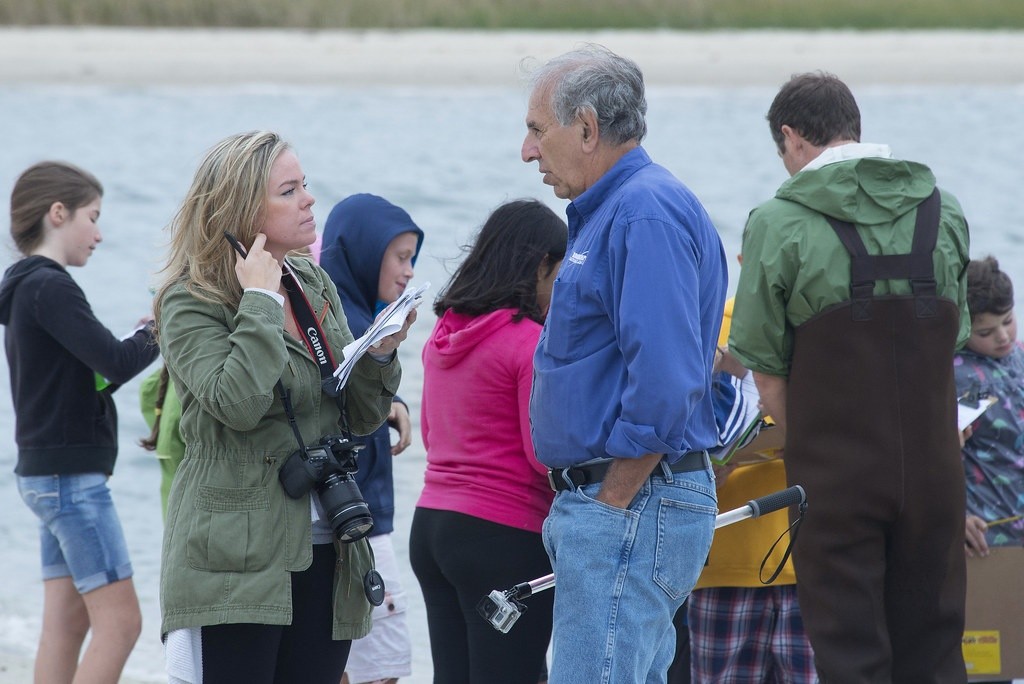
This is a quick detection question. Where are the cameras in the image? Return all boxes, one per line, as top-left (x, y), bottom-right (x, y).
top-left (475, 590), bottom-right (523, 633)
top-left (280, 432), bottom-right (375, 543)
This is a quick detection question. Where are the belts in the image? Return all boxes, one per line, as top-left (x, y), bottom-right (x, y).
top-left (547, 451), bottom-right (707, 491)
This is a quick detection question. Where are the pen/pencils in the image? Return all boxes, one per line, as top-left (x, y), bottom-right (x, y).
top-left (222, 229), bottom-right (247, 260)
top-left (553, 276), bottom-right (561, 283)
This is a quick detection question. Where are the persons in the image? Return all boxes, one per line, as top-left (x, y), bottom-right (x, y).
top-left (134, 130), bottom-right (425, 684)
top-left (0, 159), bottom-right (160, 684)
top-left (407, 41), bottom-right (1024, 684)
